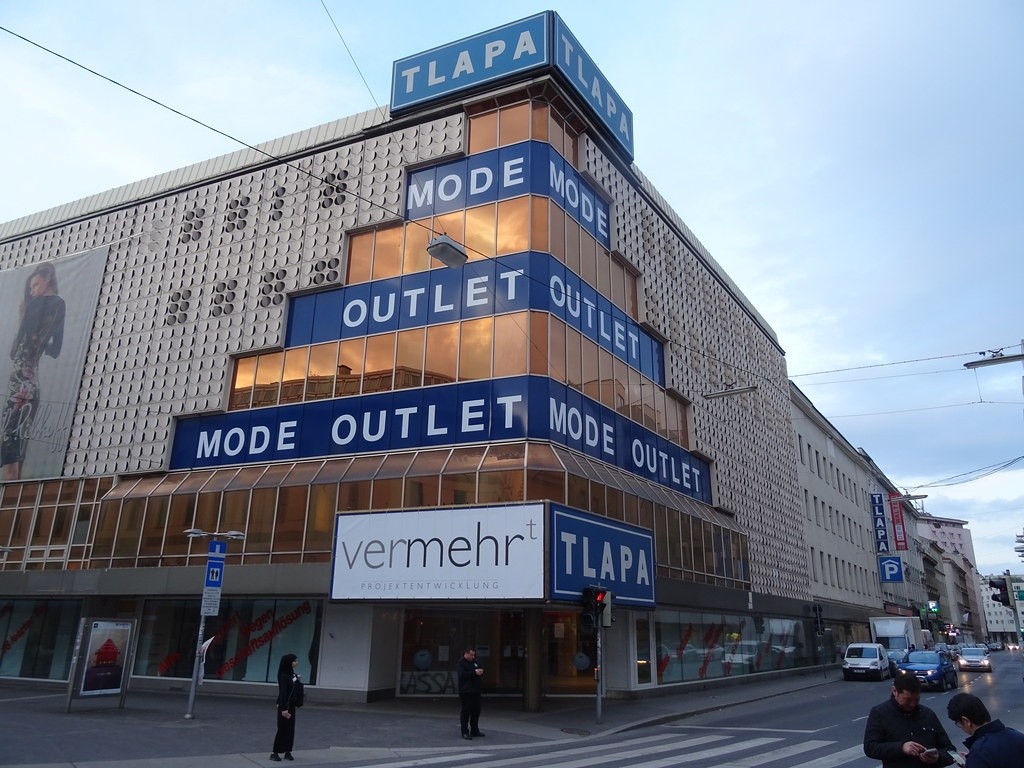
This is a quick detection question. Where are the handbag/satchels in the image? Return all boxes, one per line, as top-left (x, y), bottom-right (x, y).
top-left (468, 669), bottom-right (487, 693)
top-left (294, 673), bottom-right (305, 708)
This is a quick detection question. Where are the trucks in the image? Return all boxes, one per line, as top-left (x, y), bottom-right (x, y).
top-left (869, 616), bottom-right (924, 676)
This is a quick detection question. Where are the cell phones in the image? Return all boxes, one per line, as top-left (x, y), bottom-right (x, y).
top-left (947, 750), bottom-right (965, 765)
top-left (925, 748), bottom-right (937, 753)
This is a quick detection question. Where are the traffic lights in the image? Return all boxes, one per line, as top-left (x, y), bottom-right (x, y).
top-left (989, 576), bottom-right (1015, 607)
top-left (580, 585), bottom-right (607, 641)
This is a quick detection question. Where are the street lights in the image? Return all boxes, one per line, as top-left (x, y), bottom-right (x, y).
top-left (183, 529), bottom-right (246, 721)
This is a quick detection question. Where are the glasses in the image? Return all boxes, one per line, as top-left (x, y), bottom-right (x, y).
top-left (952, 719), bottom-right (964, 730)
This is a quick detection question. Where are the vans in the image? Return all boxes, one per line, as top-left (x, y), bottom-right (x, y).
top-left (840, 643), bottom-right (891, 681)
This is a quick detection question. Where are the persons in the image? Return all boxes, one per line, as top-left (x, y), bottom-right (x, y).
top-left (270, 654), bottom-right (303, 761)
top-left (947, 693), bottom-right (1024, 768)
top-left (864, 672), bottom-right (956, 768)
top-left (457, 647), bottom-right (485, 740)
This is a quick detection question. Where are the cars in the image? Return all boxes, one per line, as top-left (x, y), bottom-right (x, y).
top-left (934, 642), bottom-right (1005, 661)
top-left (957, 648), bottom-right (993, 673)
top-left (892, 649), bottom-right (958, 692)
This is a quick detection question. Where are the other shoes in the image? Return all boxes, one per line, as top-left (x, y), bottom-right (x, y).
top-left (461, 729), bottom-right (472, 741)
top-left (284, 753), bottom-right (295, 761)
top-left (269, 753), bottom-right (282, 763)
top-left (470, 727), bottom-right (486, 737)
